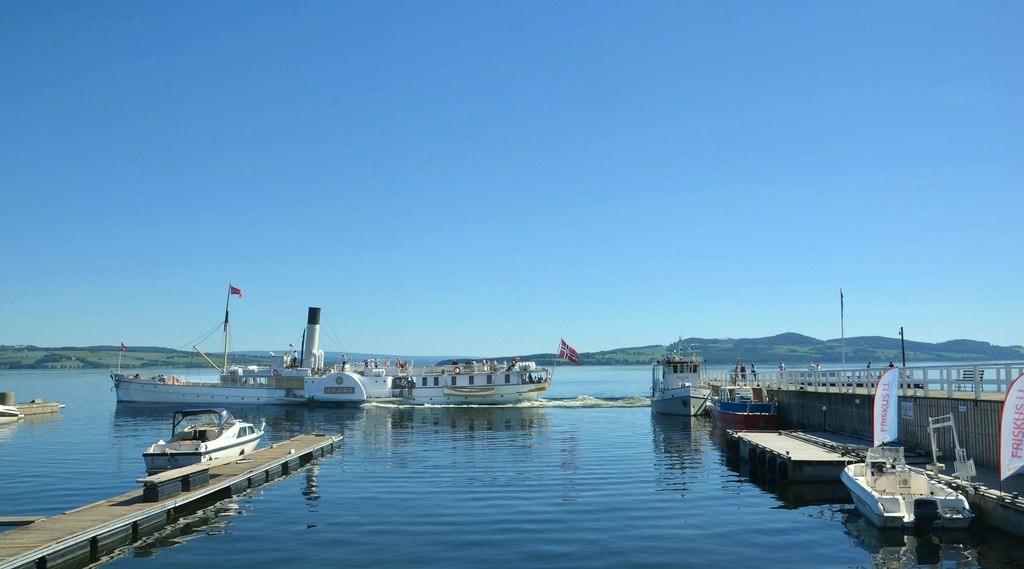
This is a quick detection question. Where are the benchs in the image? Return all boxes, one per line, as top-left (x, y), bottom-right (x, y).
top-left (135, 455), bottom-right (242, 501)
top-left (953, 370), bottom-right (984, 391)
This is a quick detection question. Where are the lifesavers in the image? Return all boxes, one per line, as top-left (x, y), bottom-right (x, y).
top-left (454, 366), bottom-right (461, 374)
top-left (273, 369), bottom-right (278, 376)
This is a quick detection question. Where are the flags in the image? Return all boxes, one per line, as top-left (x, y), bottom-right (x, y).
top-left (560, 341), bottom-right (580, 363)
top-left (120, 343), bottom-right (126, 351)
top-left (230, 286), bottom-right (243, 296)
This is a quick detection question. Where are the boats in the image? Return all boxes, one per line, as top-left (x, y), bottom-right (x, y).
top-left (840, 447), bottom-right (976, 531)
top-left (706, 357), bottom-right (786, 430)
top-left (650, 336), bottom-right (713, 416)
top-left (0, 405), bottom-right (25, 425)
top-left (110, 282), bottom-right (551, 404)
top-left (142, 408), bottom-right (265, 475)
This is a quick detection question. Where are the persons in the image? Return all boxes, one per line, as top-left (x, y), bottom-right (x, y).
top-left (506, 358), bottom-right (517, 371)
top-left (815, 364), bottom-right (821, 370)
top-left (395, 358), bottom-right (408, 370)
top-left (891, 457), bottom-right (898, 469)
top-left (482, 357), bottom-right (497, 371)
top-left (284, 350), bottom-right (297, 367)
top-left (751, 363), bottom-right (756, 379)
top-left (452, 359), bottom-right (458, 365)
top-left (465, 359), bottom-right (476, 372)
top-left (527, 373), bottom-right (543, 383)
top-left (867, 361), bottom-right (872, 368)
top-left (341, 355), bottom-right (347, 371)
top-left (889, 362), bottom-right (893, 367)
top-left (807, 362), bottom-right (816, 370)
top-left (386, 359), bottom-right (389, 367)
top-left (156, 375), bottom-right (166, 384)
top-left (364, 357), bottom-right (380, 368)
top-left (734, 363), bottom-right (747, 381)
top-left (779, 361), bottom-right (786, 371)
